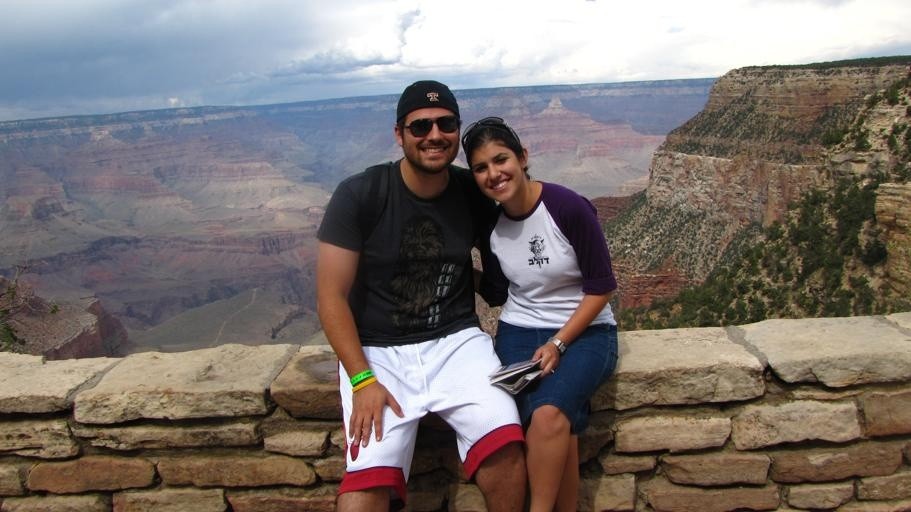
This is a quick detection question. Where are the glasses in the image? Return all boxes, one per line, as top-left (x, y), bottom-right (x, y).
top-left (403, 115), bottom-right (460, 137)
top-left (461, 116), bottom-right (520, 149)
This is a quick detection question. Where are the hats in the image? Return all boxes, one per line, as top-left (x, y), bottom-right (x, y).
top-left (396, 80), bottom-right (459, 122)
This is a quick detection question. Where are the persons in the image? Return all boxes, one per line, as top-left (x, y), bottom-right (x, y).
top-left (312, 77), bottom-right (529, 510)
top-left (462, 116), bottom-right (619, 512)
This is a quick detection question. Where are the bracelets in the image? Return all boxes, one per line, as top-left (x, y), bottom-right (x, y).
top-left (351, 376), bottom-right (377, 393)
top-left (351, 368), bottom-right (373, 386)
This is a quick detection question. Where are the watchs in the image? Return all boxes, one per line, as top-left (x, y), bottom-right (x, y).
top-left (547, 335), bottom-right (566, 354)
